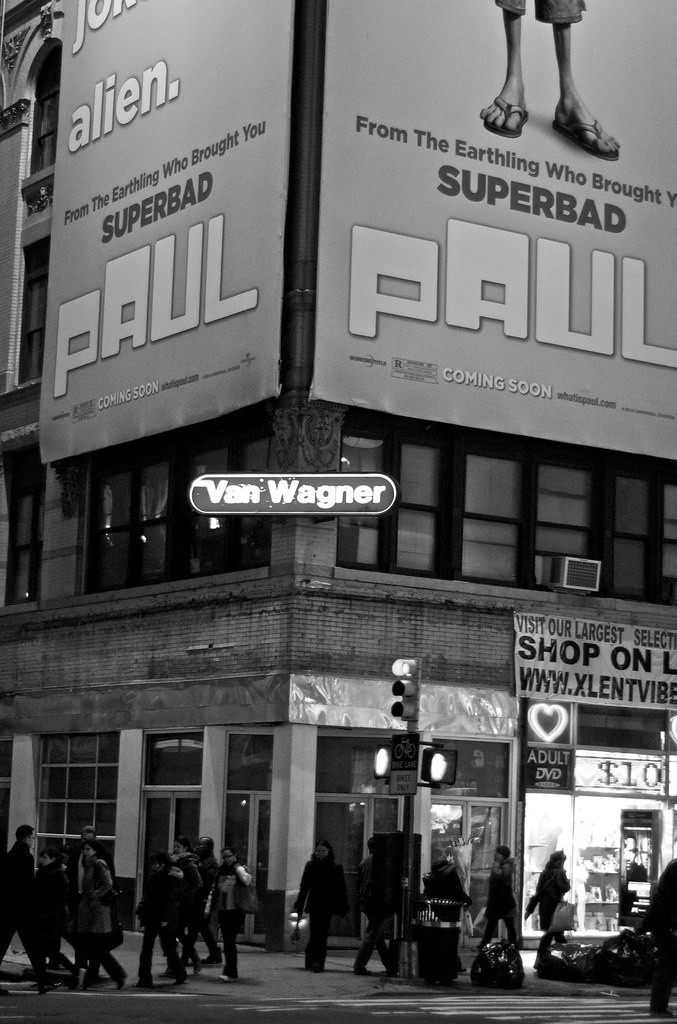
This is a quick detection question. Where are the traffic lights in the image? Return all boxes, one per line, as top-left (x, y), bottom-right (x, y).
top-left (392, 659), bottom-right (420, 722)
top-left (373, 744), bottom-right (392, 779)
top-left (419, 747), bottom-right (456, 785)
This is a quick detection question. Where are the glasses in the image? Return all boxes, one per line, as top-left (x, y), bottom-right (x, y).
top-left (223, 854), bottom-right (234, 859)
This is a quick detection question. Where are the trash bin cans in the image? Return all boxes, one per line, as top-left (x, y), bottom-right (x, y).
top-left (411, 895), bottom-right (462, 982)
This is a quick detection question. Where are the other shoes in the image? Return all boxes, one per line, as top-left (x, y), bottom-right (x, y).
top-left (134, 979), bottom-right (153, 989)
top-left (173, 975), bottom-right (188, 984)
top-left (193, 962), bottom-right (202, 974)
top-left (38, 981), bottom-right (64, 994)
top-left (218, 974), bottom-right (238, 983)
top-left (161, 969), bottom-right (175, 979)
top-left (116, 972), bottom-right (127, 990)
top-left (650, 1009), bottom-right (674, 1018)
top-left (386, 973), bottom-right (400, 978)
top-left (76, 968), bottom-right (87, 987)
top-left (201, 957), bottom-right (222, 964)
top-left (354, 967), bottom-right (373, 975)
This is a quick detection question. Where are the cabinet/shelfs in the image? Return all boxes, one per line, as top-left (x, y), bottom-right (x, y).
top-left (529, 843), bottom-right (619, 931)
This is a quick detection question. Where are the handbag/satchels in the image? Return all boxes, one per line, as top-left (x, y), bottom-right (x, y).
top-left (93, 860), bottom-right (123, 906)
top-left (233, 867), bottom-right (259, 914)
top-left (548, 889), bottom-right (575, 932)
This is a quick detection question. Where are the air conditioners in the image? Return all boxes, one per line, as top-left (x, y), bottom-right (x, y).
top-left (544, 556), bottom-right (602, 591)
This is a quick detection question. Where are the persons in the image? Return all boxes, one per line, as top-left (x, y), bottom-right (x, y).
top-left (353, 838), bottom-right (397, 975)
top-left (480, 1), bottom-right (621, 161)
top-left (525, 851), bottom-right (571, 970)
top-left (293, 842), bottom-right (351, 972)
top-left (469, 846), bottom-right (520, 952)
top-left (635, 860), bottom-right (677, 1019)
top-left (619, 832), bottom-right (648, 927)
top-left (133, 835), bottom-right (252, 989)
top-left (422, 848), bottom-right (472, 972)
top-left (0, 825), bottom-right (127, 994)
